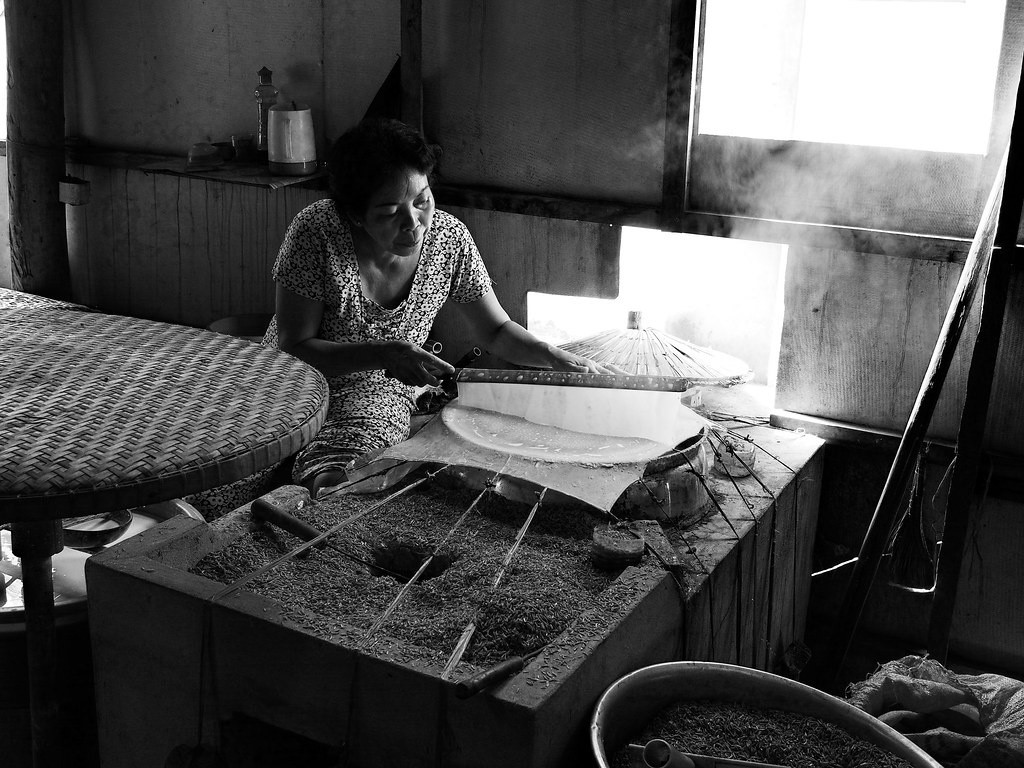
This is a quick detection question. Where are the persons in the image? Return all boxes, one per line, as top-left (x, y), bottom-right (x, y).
top-left (131, 116), bottom-right (635, 523)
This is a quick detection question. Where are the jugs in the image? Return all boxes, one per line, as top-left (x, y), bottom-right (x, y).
top-left (269, 103), bottom-right (317, 175)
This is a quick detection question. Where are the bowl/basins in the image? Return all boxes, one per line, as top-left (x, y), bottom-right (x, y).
top-left (206, 315), bottom-right (270, 342)
top-left (187, 143), bottom-right (219, 166)
top-left (62, 509), bottom-right (133, 548)
top-left (592, 662), bottom-right (944, 768)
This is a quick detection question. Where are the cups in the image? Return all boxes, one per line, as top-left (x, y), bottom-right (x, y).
top-left (231, 134), bottom-right (253, 157)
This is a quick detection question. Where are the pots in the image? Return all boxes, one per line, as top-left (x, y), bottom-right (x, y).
top-left (442, 399), bottom-right (707, 522)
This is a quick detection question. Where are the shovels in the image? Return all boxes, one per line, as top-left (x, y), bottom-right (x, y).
top-left (626, 736), bottom-right (796, 768)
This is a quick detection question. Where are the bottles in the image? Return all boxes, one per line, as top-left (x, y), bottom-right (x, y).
top-left (254, 67), bottom-right (278, 151)
